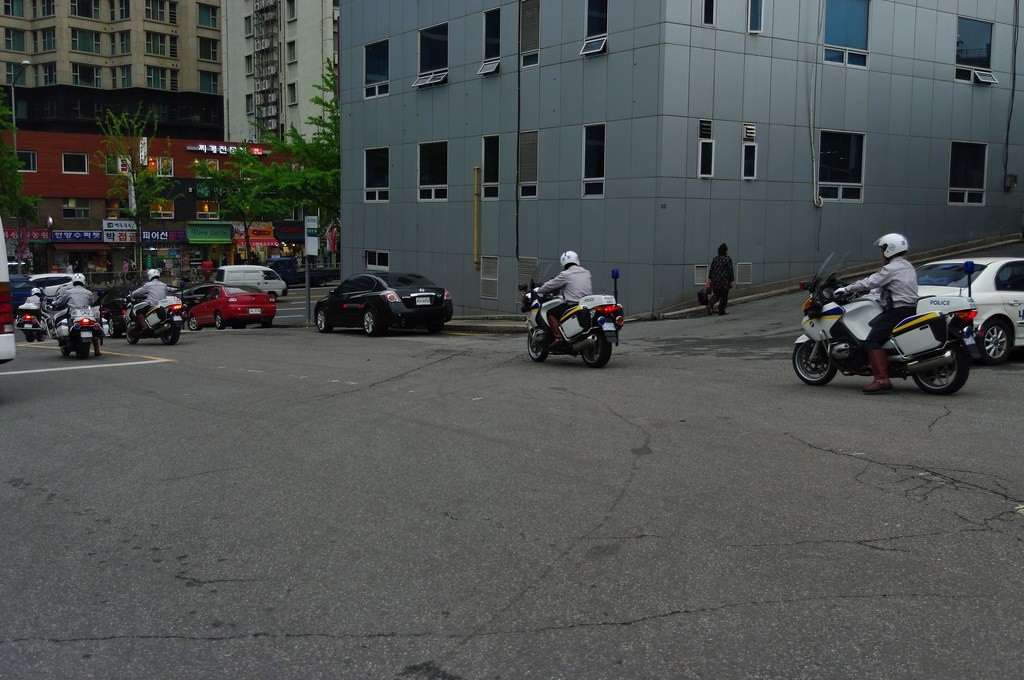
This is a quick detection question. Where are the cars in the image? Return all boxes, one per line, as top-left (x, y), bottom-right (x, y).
top-left (866, 257), bottom-right (1023, 364)
top-left (174, 284), bottom-right (222, 319)
top-left (91, 285), bottom-right (143, 337)
top-left (28, 273), bottom-right (76, 303)
top-left (9, 274), bottom-right (37, 317)
top-left (188, 284), bottom-right (277, 331)
top-left (314, 272), bottom-right (453, 336)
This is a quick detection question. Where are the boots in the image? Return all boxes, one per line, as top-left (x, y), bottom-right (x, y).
top-left (136, 314), bottom-right (148, 335)
top-left (92, 338), bottom-right (102, 356)
top-left (861, 349), bottom-right (894, 392)
top-left (547, 315), bottom-right (563, 347)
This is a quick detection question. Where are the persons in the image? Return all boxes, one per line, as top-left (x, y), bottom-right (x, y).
top-left (123, 258), bottom-right (137, 282)
top-left (52, 273), bottom-right (102, 357)
top-left (251, 251), bottom-right (261, 262)
top-left (106, 260), bottom-right (113, 284)
top-left (233, 251), bottom-right (241, 265)
top-left (26, 287), bottom-right (51, 325)
top-left (295, 251), bottom-right (302, 268)
top-left (221, 253), bottom-right (227, 266)
top-left (533, 251), bottom-right (593, 349)
top-left (128, 269), bottom-right (167, 334)
top-left (66, 260), bottom-right (82, 274)
top-left (832, 233), bottom-right (919, 392)
top-left (706, 243), bottom-right (734, 315)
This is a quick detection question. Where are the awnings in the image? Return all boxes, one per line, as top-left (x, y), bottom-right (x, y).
top-left (233, 238), bottom-right (279, 246)
top-left (52, 243), bottom-right (111, 250)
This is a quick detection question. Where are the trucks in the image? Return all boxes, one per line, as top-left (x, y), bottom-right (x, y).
top-left (266, 256), bottom-right (341, 286)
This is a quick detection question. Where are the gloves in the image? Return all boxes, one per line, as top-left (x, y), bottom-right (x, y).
top-left (533, 287), bottom-right (540, 294)
top-left (52, 301), bottom-right (56, 305)
top-left (126, 295), bottom-right (133, 297)
top-left (834, 287), bottom-right (849, 296)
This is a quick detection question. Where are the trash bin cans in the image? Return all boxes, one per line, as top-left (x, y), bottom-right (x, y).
top-left (201, 261), bottom-right (213, 283)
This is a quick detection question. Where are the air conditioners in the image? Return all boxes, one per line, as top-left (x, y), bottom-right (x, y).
top-left (254, 0), bottom-right (278, 129)
top-left (248, 139), bottom-right (258, 143)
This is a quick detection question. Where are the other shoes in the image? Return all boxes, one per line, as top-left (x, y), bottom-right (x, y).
top-left (719, 311), bottom-right (729, 315)
top-left (706, 305), bottom-right (713, 315)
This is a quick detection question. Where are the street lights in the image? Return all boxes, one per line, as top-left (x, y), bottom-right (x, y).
top-left (10, 59), bottom-right (33, 272)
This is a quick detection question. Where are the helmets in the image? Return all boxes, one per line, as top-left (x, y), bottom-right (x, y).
top-left (877, 233), bottom-right (908, 258)
top-left (559, 251), bottom-right (579, 268)
top-left (147, 268), bottom-right (161, 281)
top-left (32, 287), bottom-right (41, 296)
top-left (72, 273), bottom-right (86, 286)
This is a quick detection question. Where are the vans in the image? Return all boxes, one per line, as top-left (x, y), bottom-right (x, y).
top-left (215, 266), bottom-right (289, 300)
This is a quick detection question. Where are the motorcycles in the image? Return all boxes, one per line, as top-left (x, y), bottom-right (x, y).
top-left (791, 248), bottom-right (978, 394)
top-left (520, 258), bottom-right (625, 367)
top-left (15, 302), bottom-right (53, 342)
top-left (45, 305), bottom-right (108, 359)
top-left (118, 289), bottom-right (184, 344)
